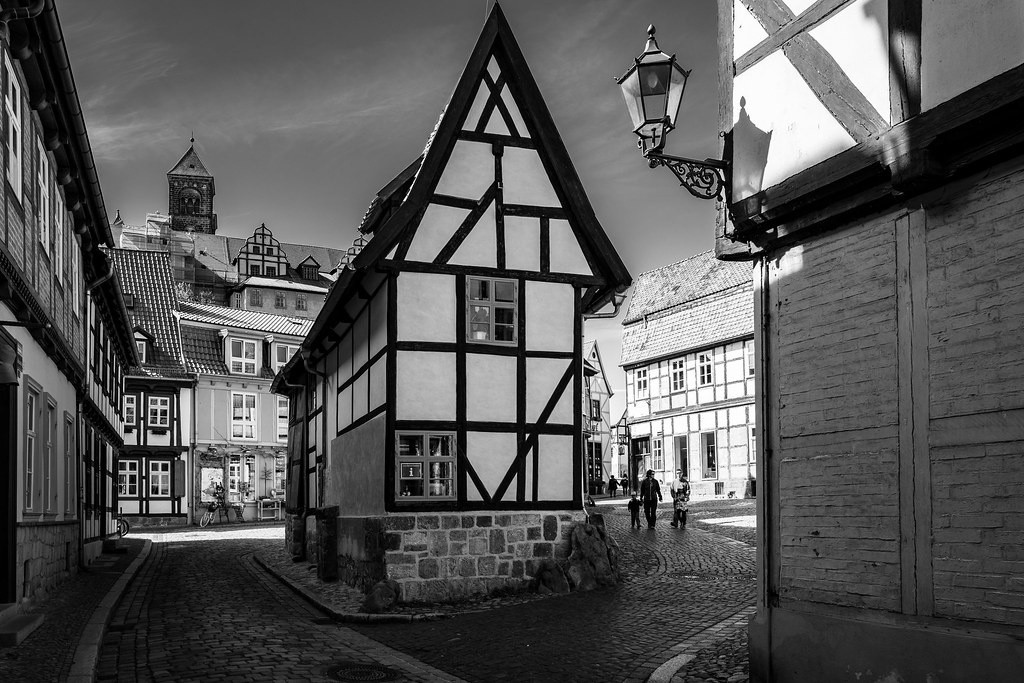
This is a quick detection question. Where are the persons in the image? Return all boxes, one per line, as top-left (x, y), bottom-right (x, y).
top-left (627, 491), bottom-right (642, 530)
top-left (608, 475), bottom-right (619, 497)
top-left (670, 468), bottom-right (690, 530)
top-left (640, 470), bottom-right (662, 530)
top-left (620, 474), bottom-right (628, 497)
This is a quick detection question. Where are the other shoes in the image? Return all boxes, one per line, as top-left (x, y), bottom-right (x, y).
top-left (680, 525), bottom-right (685, 530)
top-left (637, 527), bottom-right (641, 530)
top-left (648, 526), bottom-right (656, 530)
top-left (671, 522), bottom-right (678, 528)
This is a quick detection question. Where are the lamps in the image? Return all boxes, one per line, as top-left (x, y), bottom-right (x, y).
top-left (617, 24), bottom-right (733, 218)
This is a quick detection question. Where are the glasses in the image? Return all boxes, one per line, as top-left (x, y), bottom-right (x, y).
top-left (651, 473), bottom-right (654, 475)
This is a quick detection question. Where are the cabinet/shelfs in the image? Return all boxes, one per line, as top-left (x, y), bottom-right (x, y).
top-left (257, 499), bottom-right (281, 521)
